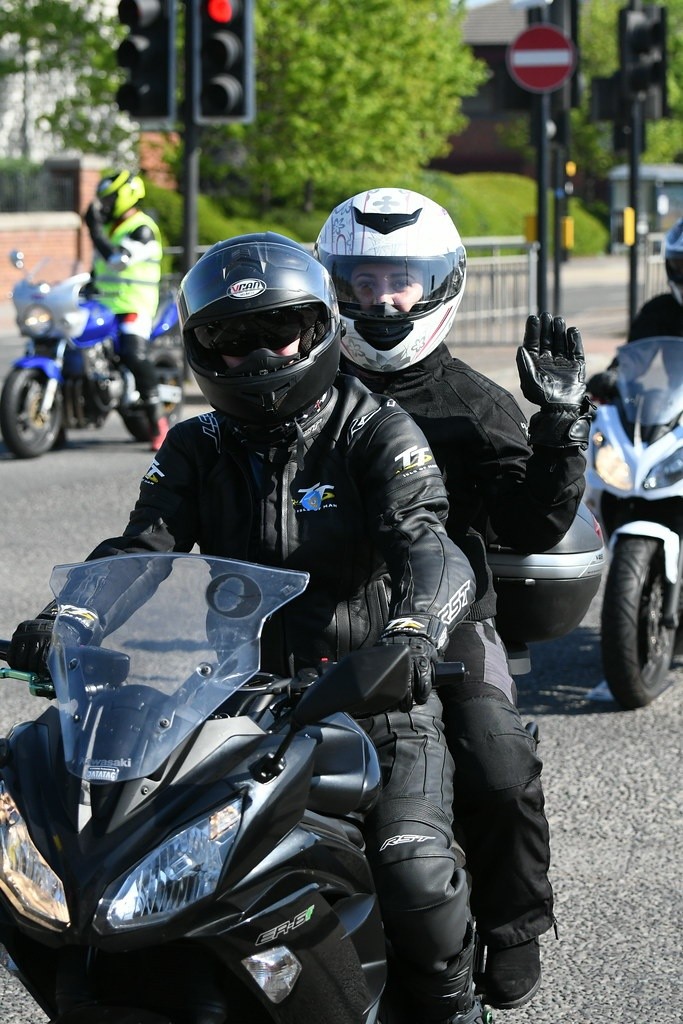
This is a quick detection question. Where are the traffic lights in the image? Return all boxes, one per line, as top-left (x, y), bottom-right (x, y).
top-left (193, 0), bottom-right (256, 124)
top-left (116, 0), bottom-right (177, 128)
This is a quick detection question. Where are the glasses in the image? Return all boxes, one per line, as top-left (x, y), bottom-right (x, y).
top-left (194, 307), bottom-right (318, 355)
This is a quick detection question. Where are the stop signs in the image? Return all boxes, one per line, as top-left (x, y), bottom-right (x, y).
top-left (507, 23), bottom-right (575, 93)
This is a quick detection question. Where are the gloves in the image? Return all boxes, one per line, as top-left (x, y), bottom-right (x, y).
top-left (7, 597), bottom-right (99, 677)
top-left (84, 201), bottom-right (101, 232)
top-left (586, 364), bottom-right (619, 403)
top-left (373, 613), bottom-right (449, 713)
top-left (515, 313), bottom-right (597, 451)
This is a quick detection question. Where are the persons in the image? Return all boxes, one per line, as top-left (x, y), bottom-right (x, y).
top-left (0, 232), bottom-right (478, 1024)
top-left (582, 219), bottom-right (683, 406)
top-left (307, 187), bottom-right (594, 1008)
top-left (72, 172), bottom-right (170, 453)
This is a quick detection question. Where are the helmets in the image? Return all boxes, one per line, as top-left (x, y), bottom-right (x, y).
top-left (96, 169), bottom-right (146, 224)
top-left (175, 231), bottom-right (346, 423)
top-left (313, 188), bottom-right (467, 373)
top-left (665, 218), bottom-right (683, 305)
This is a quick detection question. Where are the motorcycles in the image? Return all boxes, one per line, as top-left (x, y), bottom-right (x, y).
top-left (0, 248), bottom-right (191, 459)
top-left (0, 551), bottom-right (499, 1024)
top-left (574, 337), bottom-right (683, 710)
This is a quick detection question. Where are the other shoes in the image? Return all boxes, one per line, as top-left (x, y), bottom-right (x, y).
top-left (147, 416), bottom-right (169, 451)
top-left (483, 937), bottom-right (542, 1008)
